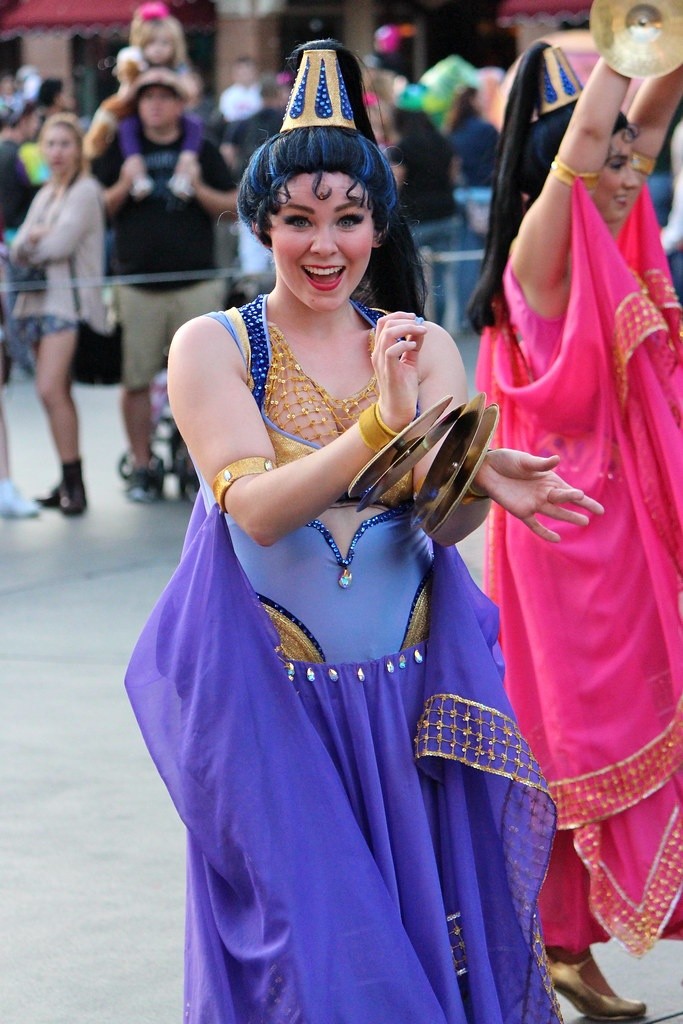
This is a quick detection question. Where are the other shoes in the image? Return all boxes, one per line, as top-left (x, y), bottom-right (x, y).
top-left (60, 466), bottom-right (86, 512)
top-left (549, 948), bottom-right (646, 1021)
top-left (128, 465), bottom-right (158, 504)
top-left (167, 171), bottom-right (196, 201)
top-left (130, 174), bottom-right (152, 200)
top-left (178, 471), bottom-right (202, 500)
top-left (35, 487), bottom-right (62, 507)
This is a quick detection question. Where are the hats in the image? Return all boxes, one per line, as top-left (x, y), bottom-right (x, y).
top-left (134, 75), bottom-right (189, 103)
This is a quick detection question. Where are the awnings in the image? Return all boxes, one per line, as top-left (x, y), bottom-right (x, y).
top-left (497, 0), bottom-right (594, 28)
top-left (1, 0), bottom-right (215, 42)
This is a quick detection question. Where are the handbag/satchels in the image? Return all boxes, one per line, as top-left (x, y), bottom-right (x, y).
top-left (68, 313), bottom-right (126, 385)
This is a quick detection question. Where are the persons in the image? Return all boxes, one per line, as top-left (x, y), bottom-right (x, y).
top-left (164, 153), bottom-right (606, 1018)
top-left (0, 0), bottom-right (296, 543)
top-left (475, 42), bottom-right (683, 1024)
top-left (355, 1), bottom-right (564, 336)
